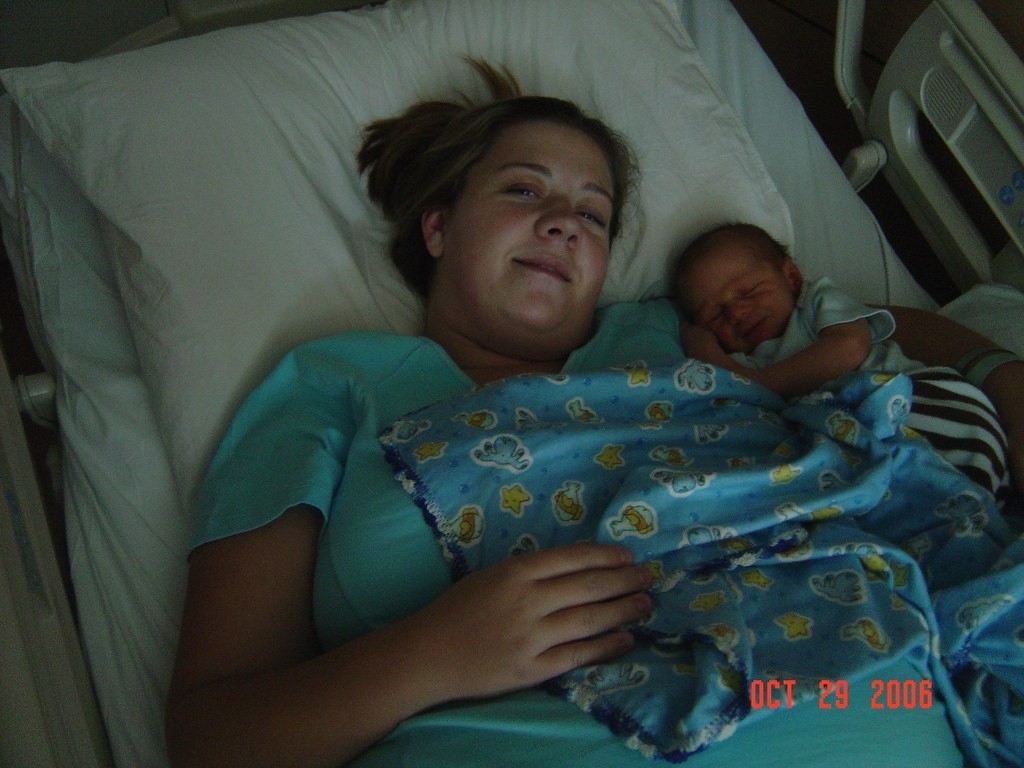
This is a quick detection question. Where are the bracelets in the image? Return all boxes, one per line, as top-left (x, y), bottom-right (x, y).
top-left (953, 347), bottom-right (1016, 374)
top-left (964, 351), bottom-right (1020, 384)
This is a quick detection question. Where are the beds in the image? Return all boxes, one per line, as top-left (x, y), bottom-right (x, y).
top-left (0, 0), bottom-right (1024, 768)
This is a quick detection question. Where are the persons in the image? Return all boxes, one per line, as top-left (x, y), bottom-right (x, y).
top-left (160, 53), bottom-right (1024, 768)
top-left (671, 219), bottom-right (1012, 512)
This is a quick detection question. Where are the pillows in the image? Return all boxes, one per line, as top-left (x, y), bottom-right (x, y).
top-left (0, 0), bottom-right (797, 528)
